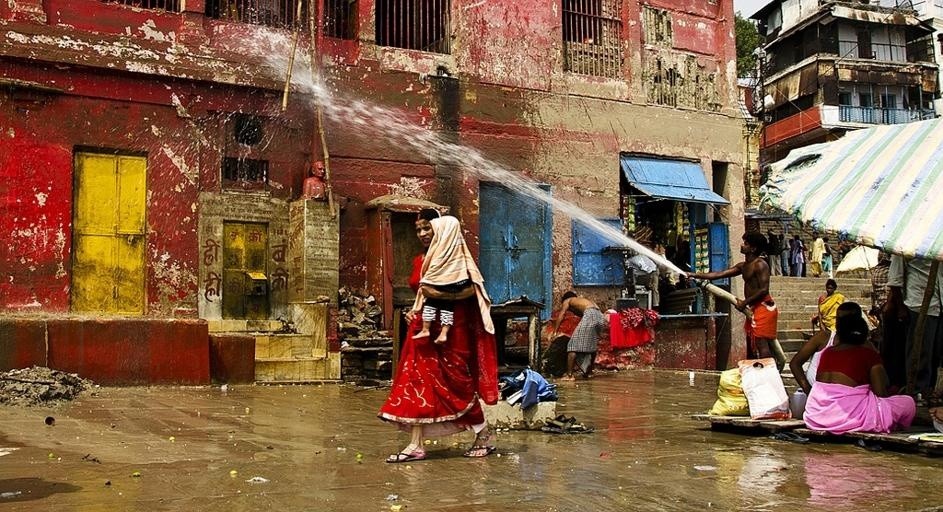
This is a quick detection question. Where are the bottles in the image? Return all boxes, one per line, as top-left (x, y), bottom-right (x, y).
top-left (792, 388), bottom-right (807, 420)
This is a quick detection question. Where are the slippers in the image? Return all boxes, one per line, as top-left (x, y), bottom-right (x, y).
top-left (385, 448), bottom-right (427, 462)
top-left (461, 444), bottom-right (496, 458)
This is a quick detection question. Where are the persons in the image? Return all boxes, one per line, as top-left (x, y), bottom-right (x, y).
top-left (303, 161), bottom-right (328, 200)
top-left (377, 208), bottom-right (498, 463)
top-left (759, 230), bottom-right (854, 277)
top-left (790, 249), bottom-right (943, 433)
top-left (686, 229), bottom-right (778, 358)
top-left (551, 291), bottom-right (605, 381)
top-left (625, 223), bottom-right (690, 312)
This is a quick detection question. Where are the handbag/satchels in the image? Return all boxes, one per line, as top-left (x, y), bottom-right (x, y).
top-left (738, 357), bottom-right (789, 420)
